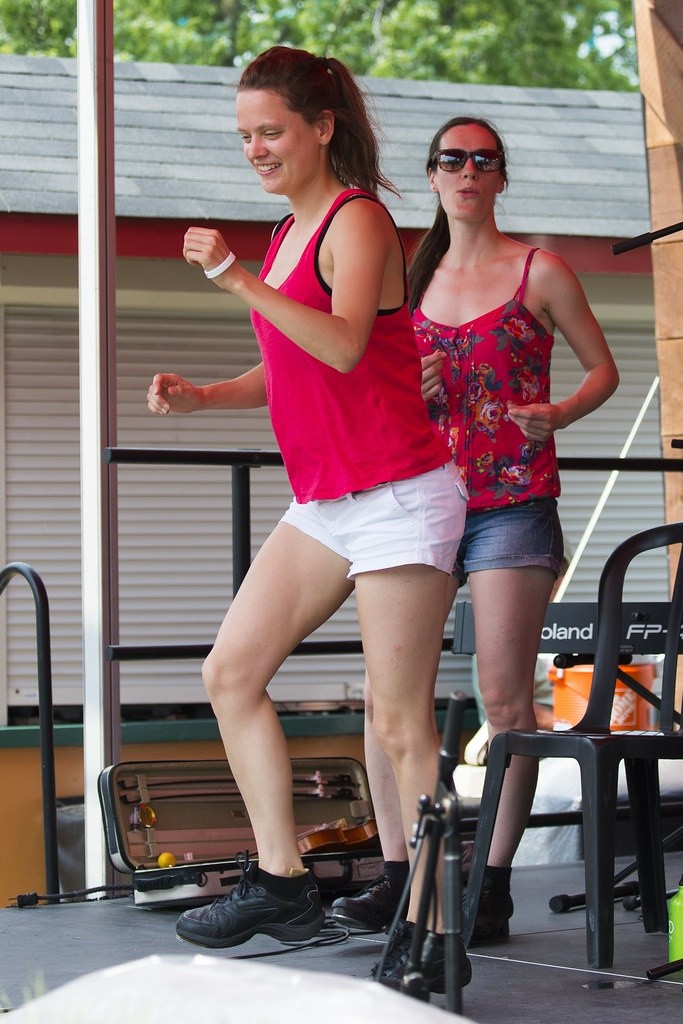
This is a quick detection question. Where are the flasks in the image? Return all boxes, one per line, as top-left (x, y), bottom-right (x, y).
top-left (668, 873), bottom-right (683, 963)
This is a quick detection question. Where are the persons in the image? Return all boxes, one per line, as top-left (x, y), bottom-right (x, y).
top-left (145, 47), bottom-right (473, 996)
top-left (333, 114), bottom-right (620, 944)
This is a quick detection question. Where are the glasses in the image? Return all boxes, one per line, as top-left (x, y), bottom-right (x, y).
top-left (432, 147), bottom-right (503, 172)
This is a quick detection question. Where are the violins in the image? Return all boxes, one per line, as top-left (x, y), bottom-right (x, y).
top-left (136, 815), bottom-right (379, 872)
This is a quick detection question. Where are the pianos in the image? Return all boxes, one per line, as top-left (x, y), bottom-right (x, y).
top-left (449, 600), bottom-right (683, 990)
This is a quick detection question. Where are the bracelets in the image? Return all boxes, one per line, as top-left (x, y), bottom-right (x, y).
top-left (204, 252), bottom-right (236, 279)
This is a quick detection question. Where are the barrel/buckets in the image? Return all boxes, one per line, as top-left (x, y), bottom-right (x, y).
top-left (550, 663), bottom-right (655, 732)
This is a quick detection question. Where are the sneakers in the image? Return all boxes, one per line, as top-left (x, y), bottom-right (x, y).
top-left (362, 922), bottom-right (472, 994)
top-left (461, 877), bottom-right (514, 947)
top-left (176, 850), bottom-right (326, 950)
top-left (332, 875), bottom-right (401, 936)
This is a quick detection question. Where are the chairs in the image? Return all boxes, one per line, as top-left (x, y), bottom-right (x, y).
top-left (464, 523), bottom-right (683, 969)
